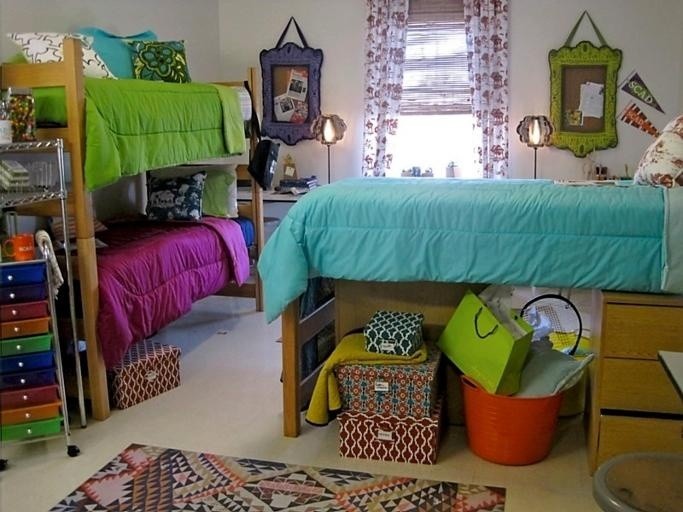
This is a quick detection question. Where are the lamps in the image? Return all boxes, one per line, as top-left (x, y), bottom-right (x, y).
top-left (310, 113), bottom-right (347, 184)
top-left (516, 114), bottom-right (553, 179)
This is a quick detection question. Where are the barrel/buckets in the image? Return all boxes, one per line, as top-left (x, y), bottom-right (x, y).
top-left (460, 372), bottom-right (565, 466)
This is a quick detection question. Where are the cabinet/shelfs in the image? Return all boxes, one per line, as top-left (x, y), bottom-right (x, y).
top-left (588, 292), bottom-right (683, 477)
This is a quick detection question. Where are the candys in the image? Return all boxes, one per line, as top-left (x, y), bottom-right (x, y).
top-left (6, 94), bottom-right (37, 142)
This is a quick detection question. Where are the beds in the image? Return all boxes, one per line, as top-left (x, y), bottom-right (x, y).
top-left (276, 177), bottom-right (683, 438)
top-left (0, 37), bottom-right (264, 422)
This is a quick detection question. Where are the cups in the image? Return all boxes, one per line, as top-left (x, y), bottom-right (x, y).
top-left (2, 233), bottom-right (35, 262)
top-left (0, 121), bottom-right (12, 144)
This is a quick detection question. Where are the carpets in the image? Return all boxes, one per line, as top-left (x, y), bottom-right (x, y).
top-left (51, 443), bottom-right (507, 512)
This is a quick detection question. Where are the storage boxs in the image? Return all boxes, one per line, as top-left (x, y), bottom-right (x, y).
top-left (332, 310), bottom-right (450, 463)
top-left (108, 339), bottom-right (182, 410)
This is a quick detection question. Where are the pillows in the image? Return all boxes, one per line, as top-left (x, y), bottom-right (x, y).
top-left (143, 162), bottom-right (241, 226)
top-left (632, 114), bottom-right (683, 188)
top-left (48, 215), bottom-right (108, 242)
top-left (5, 24), bottom-right (193, 83)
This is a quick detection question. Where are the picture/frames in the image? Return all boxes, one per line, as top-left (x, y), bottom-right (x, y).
top-left (259, 42), bottom-right (324, 147)
top-left (547, 41), bottom-right (624, 159)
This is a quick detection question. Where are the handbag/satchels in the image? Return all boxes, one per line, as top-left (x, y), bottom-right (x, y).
top-left (247, 140), bottom-right (278, 189)
top-left (438, 288), bottom-right (534, 396)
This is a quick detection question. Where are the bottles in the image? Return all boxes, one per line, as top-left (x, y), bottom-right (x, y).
top-left (7, 86), bottom-right (37, 142)
top-left (283, 153), bottom-right (297, 180)
